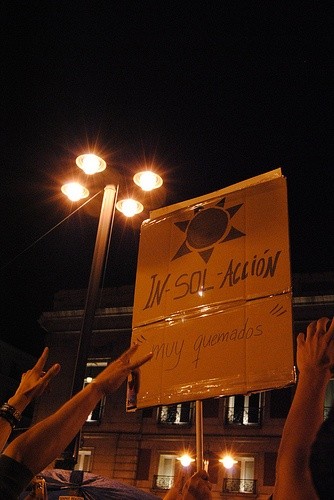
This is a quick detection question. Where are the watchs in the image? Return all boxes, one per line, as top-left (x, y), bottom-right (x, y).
top-left (0, 409), bottom-right (20, 430)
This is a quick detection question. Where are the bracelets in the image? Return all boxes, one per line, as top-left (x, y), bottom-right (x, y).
top-left (2, 403), bottom-right (20, 420)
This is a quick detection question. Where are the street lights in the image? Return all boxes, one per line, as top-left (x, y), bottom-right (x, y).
top-left (55, 150), bottom-right (165, 472)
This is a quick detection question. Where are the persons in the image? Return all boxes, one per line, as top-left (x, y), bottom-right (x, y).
top-left (0, 317), bottom-right (334, 500)
